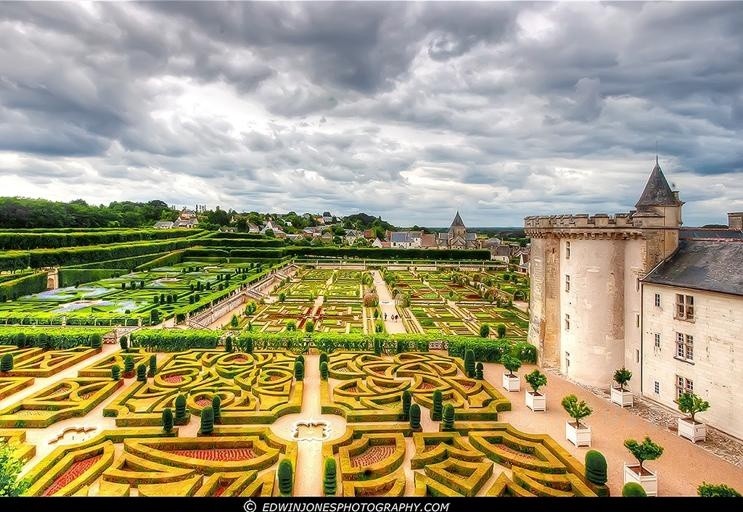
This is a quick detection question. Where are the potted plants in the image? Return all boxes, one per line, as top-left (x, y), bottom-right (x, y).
top-left (673, 389), bottom-right (710, 445)
top-left (611, 368), bottom-right (634, 408)
top-left (561, 394), bottom-right (593, 448)
top-left (500, 353), bottom-right (522, 392)
top-left (624, 436), bottom-right (664, 498)
top-left (524, 369), bottom-right (548, 411)
